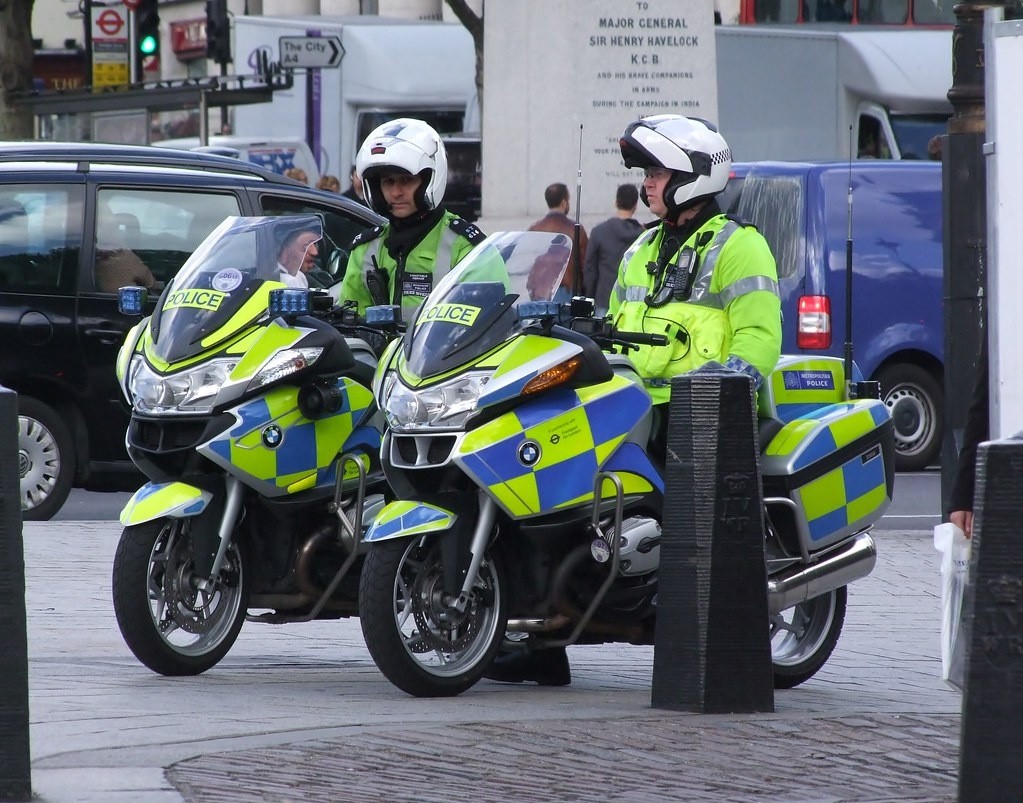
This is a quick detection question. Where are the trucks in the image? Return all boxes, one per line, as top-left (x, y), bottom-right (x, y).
top-left (715, 27), bottom-right (955, 162)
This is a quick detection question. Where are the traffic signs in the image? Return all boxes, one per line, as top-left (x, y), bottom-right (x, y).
top-left (279, 36), bottom-right (346, 69)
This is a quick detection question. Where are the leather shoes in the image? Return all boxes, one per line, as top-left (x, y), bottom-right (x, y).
top-left (481, 645), bottom-right (572, 686)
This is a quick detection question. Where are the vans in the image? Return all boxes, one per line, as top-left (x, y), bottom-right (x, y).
top-left (154, 136), bottom-right (323, 192)
top-left (724, 157), bottom-right (953, 472)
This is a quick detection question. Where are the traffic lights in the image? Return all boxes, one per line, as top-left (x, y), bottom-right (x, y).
top-left (136, 0), bottom-right (161, 59)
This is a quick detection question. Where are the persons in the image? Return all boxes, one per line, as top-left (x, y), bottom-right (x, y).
top-left (274, 229), bottom-right (320, 289)
top-left (342, 164), bottom-right (370, 210)
top-left (315, 174), bottom-right (341, 195)
top-left (583, 183), bottom-right (646, 321)
top-left (485, 111), bottom-right (783, 687)
top-left (333, 118), bottom-right (510, 334)
top-left (284, 167), bottom-right (308, 186)
top-left (527, 182), bottom-right (589, 306)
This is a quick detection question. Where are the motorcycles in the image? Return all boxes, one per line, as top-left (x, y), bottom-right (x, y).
top-left (357, 229), bottom-right (899, 699)
top-left (113, 210), bottom-right (401, 679)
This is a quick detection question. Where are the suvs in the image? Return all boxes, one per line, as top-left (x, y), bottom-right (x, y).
top-left (442, 137), bottom-right (481, 220)
top-left (0, 149), bottom-right (393, 524)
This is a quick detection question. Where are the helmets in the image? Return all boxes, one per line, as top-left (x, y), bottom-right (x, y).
top-left (355, 116), bottom-right (448, 227)
top-left (618, 115), bottom-right (733, 217)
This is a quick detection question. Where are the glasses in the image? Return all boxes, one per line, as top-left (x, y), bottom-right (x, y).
top-left (644, 165), bottom-right (675, 181)
